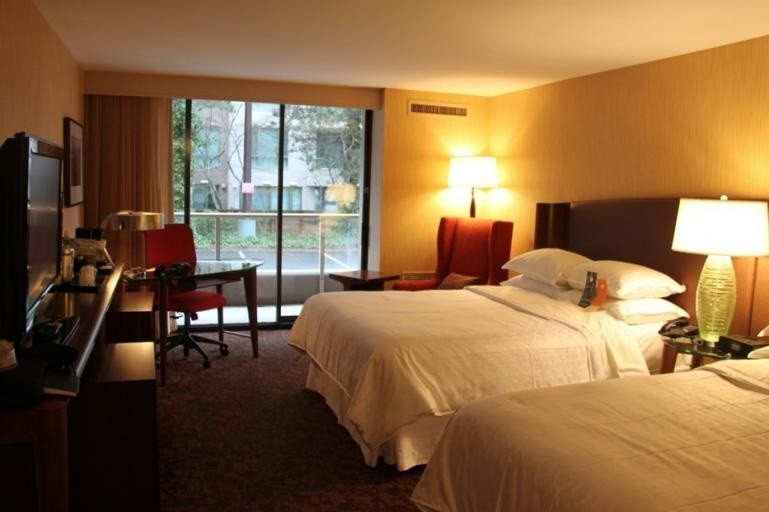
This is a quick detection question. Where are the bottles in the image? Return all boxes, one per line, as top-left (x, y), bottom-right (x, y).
top-left (62, 247), bottom-right (75, 282)
top-left (78, 265), bottom-right (98, 287)
top-left (0, 339), bottom-right (18, 373)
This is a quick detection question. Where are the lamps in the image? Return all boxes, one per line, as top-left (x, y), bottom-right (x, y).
top-left (448, 157), bottom-right (496, 217)
top-left (670, 194), bottom-right (769, 341)
top-left (99, 210), bottom-right (165, 266)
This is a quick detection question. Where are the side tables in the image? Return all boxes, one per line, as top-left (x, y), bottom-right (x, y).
top-left (329, 270), bottom-right (400, 291)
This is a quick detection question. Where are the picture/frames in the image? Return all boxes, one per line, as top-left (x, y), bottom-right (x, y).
top-left (63, 117), bottom-right (87, 207)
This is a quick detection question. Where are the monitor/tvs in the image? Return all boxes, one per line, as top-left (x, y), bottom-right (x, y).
top-left (0, 131), bottom-right (82, 362)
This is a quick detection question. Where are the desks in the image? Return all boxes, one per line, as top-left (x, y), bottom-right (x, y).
top-left (126, 259), bottom-right (264, 386)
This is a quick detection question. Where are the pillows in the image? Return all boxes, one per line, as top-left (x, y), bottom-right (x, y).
top-left (438, 272), bottom-right (480, 290)
top-left (500, 247), bottom-right (690, 325)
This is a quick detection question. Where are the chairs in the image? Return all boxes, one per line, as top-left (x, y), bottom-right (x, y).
top-left (393, 217), bottom-right (513, 290)
top-left (142, 223), bottom-right (229, 370)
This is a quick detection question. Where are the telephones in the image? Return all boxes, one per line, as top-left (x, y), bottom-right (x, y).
top-left (659, 317), bottom-right (698, 336)
top-left (155, 262), bottom-right (193, 279)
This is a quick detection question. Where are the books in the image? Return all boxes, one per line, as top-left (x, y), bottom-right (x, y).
top-left (42, 371), bottom-right (81, 397)
